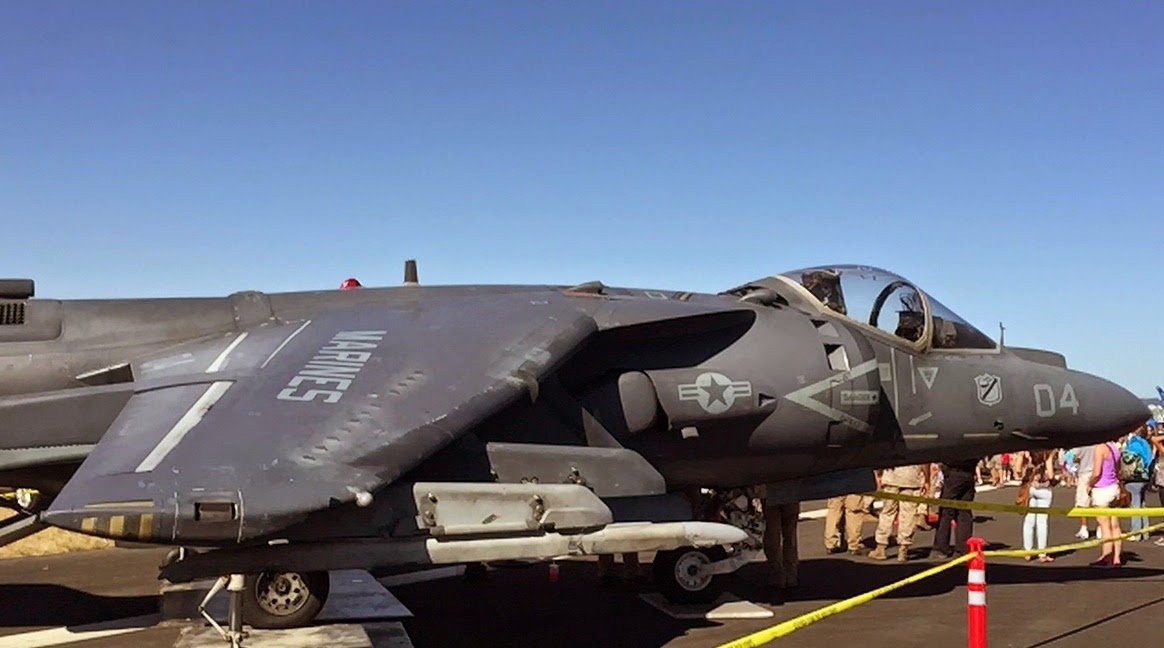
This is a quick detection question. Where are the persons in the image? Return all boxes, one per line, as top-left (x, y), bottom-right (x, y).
top-left (916, 463), bottom-right (938, 529)
top-left (927, 457), bottom-right (981, 561)
top-left (1156, 423), bottom-right (1164, 435)
top-left (761, 498), bottom-right (801, 590)
top-left (1151, 435), bottom-right (1164, 547)
top-left (598, 552), bottom-right (648, 585)
top-left (1121, 424), bottom-right (1154, 542)
top-left (1072, 446), bottom-right (1102, 540)
top-left (714, 485), bottom-right (765, 553)
top-left (863, 469), bottom-right (882, 522)
top-left (975, 448), bottom-right (1079, 488)
top-left (1146, 425), bottom-right (1155, 450)
top-left (824, 495), bottom-right (869, 554)
top-left (1016, 449), bottom-right (1058, 562)
top-left (1089, 442), bottom-right (1123, 565)
top-left (869, 464), bottom-right (929, 561)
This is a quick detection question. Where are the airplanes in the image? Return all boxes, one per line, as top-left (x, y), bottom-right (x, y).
top-left (0, 261), bottom-right (1154, 628)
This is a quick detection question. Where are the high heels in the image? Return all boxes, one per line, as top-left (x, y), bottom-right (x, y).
top-left (1089, 552), bottom-right (1113, 566)
top-left (1113, 555), bottom-right (1123, 568)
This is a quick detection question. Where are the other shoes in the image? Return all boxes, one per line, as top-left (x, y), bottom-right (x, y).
top-left (1074, 528), bottom-right (1089, 539)
top-left (868, 548), bottom-right (887, 559)
top-left (848, 549), bottom-right (862, 556)
top-left (1096, 529), bottom-right (1102, 539)
top-left (770, 580), bottom-right (798, 590)
top-left (1142, 534), bottom-right (1150, 540)
top-left (1024, 555), bottom-right (1032, 560)
top-left (1127, 534), bottom-right (1141, 541)
top-left (929, 550), bottom-right (948, 559)
top-left (916, 520), bottom-right (932, 530)
top-left (1039, 556), bottom-right (1054, 562)
top-left (828, 547), bottom-right (847, 554)
top-left (897, 547), bottom-right (908, 561)
top-left (952, 552), bottom-right (962, 559)
top-left (1154, 537), bottom-right (1164, 546)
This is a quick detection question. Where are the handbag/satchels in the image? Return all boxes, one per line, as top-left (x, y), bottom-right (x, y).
top-left (1119, 482), bottom-right (1132, 508)
top-left (1116, 450), bottom-right (1143, 481)
top-left (1015, 484), bottom-right (1030, 516)
